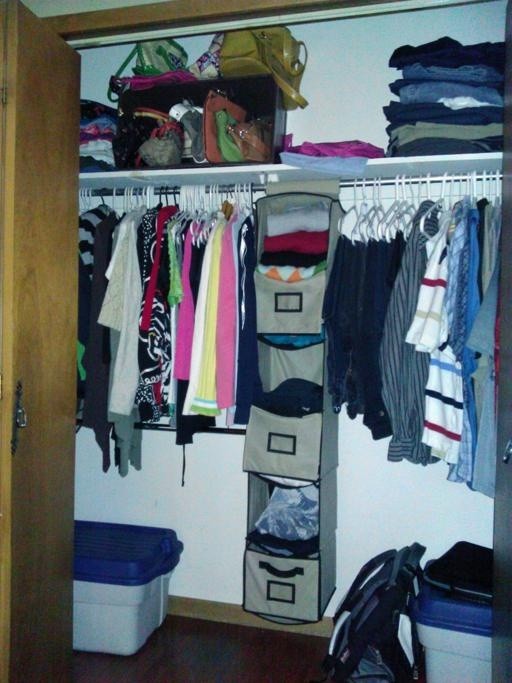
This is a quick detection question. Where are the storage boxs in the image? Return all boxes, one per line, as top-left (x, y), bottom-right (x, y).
top-left (70, 521), bottom-right (183, 658)
top-left (406, 585), bottom-right (495, 683)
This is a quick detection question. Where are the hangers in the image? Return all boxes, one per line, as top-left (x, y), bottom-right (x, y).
top-left (335, 168), bottom-right (502, 249)
top-left (81, 182), bottom-right (254, 248)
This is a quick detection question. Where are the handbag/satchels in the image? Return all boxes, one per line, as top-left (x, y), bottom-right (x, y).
top-left (108, 38), bottom-right (189, 103)
top-left (119, 92), bottom-right (270, 167)
top-left (216, 25), bottom-right (309, 110)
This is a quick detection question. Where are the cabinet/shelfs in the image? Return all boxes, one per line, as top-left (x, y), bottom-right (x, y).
top-left (79, 15), bottom-right (512, 627)
